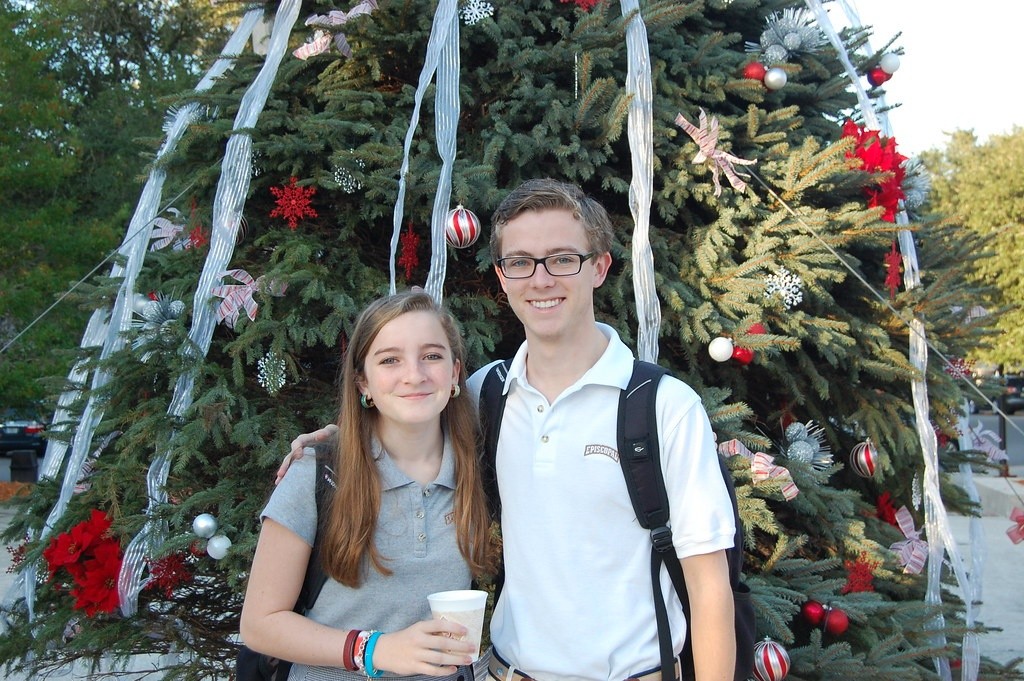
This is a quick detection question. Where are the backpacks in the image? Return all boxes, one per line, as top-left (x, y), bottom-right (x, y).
top-left (231, 425), bottom-right (344, 681)
top-left (473, 356), bottom-right (757, 680)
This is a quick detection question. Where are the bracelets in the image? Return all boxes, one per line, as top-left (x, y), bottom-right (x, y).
top-left (343, 629), bottom-right (386, 681)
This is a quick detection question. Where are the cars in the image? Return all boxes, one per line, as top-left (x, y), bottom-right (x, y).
top-left (0, 401), bottom-right (77, 457)
top-left (965, 374), bottom-right (1024, 414)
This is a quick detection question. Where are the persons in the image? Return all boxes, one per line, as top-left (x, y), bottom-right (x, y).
top-left (239, 291), bottom-right (506, 681)
top-left (275, 177), bottom-right (740, 681)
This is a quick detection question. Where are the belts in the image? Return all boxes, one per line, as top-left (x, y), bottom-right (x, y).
top-left (486, 649), bottom-right (683, 680)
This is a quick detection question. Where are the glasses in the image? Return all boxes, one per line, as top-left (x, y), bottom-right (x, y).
top-left (495, 251), bottom-right (597, 280)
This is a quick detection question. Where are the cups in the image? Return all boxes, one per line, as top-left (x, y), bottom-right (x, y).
top-left (426, 589), bottom-right (489, 664)
